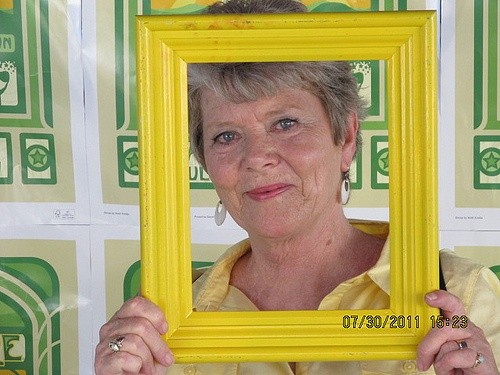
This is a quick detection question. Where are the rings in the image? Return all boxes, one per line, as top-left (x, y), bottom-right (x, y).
top-left (472, 352), bottom-right (485, 368)
top-left (108, 335), bottom-right (125, 352)
top-left (455, 339), bottom-right (469, 350)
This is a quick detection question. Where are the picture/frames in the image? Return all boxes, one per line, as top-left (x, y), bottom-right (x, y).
top-left (134, 10), bottom-right (443, 362)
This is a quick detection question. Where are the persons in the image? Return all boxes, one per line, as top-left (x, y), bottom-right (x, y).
top-left (94, 1), bottom-right (499, 374)
